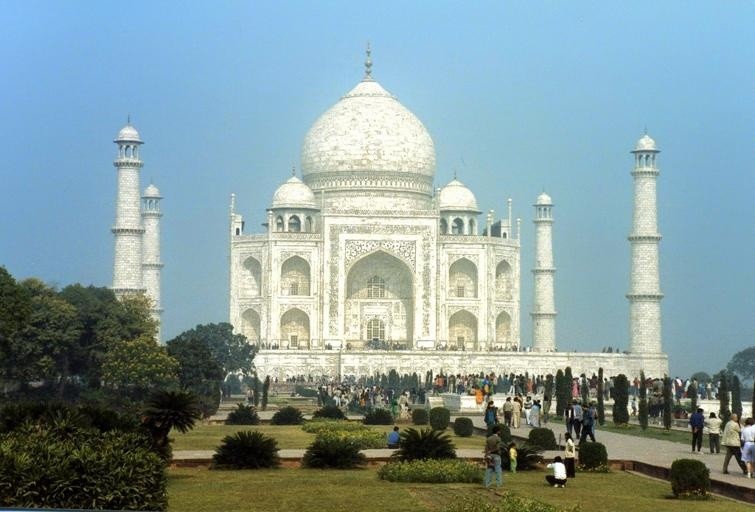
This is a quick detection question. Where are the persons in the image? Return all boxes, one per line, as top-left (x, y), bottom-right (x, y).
top-left (261, 337), bottom-right (559, 353)
top-left (601, 377), bottom-right (755, 478)
top-left (247, 388), bottom-right (255, 404)
top-left (288, 372), bottom-right (598, 490)
